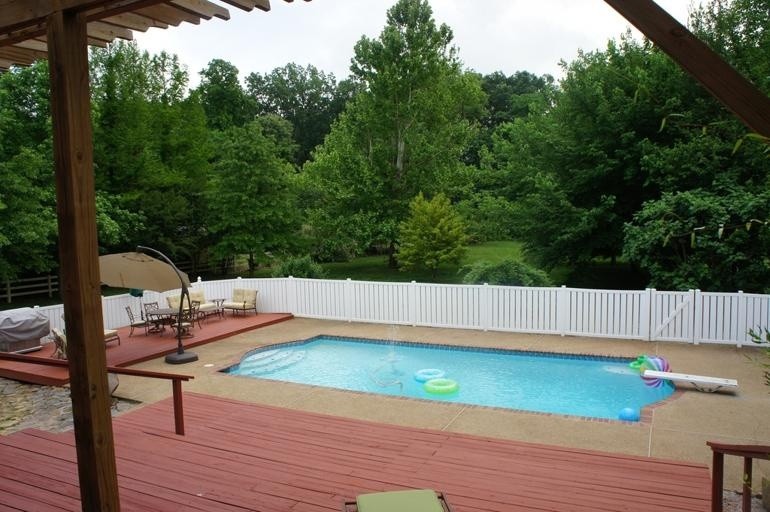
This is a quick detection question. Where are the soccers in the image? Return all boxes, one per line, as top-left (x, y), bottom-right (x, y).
top-left (640, 356), bottom-right (672, 388)
top-left (618, 408), bottom-right (639, 421)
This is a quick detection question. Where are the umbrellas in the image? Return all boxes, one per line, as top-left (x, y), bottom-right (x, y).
top-left (98, 244), bottom-right (194, 353)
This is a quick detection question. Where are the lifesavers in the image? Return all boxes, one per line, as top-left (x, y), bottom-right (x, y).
top-left (415, 368), bottom-right (444, 381)
top-left (630, 355), bottom-right (652, 368)
top-left (424, 380), bottom-right (459, 394)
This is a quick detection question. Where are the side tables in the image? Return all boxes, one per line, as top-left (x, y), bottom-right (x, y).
top-left (207, 298), bottom-right (228, 320)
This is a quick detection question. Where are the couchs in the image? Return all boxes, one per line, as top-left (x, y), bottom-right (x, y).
top-left (166, 290), bottom-right (217, 322)
top-left (216, 288), bottom-right (258, 318)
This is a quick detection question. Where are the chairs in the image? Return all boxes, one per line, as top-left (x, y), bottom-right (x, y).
top-left (344, 487), bottom-right (449, 511)
top-left (47, 300), bottom-right (202, 361)
